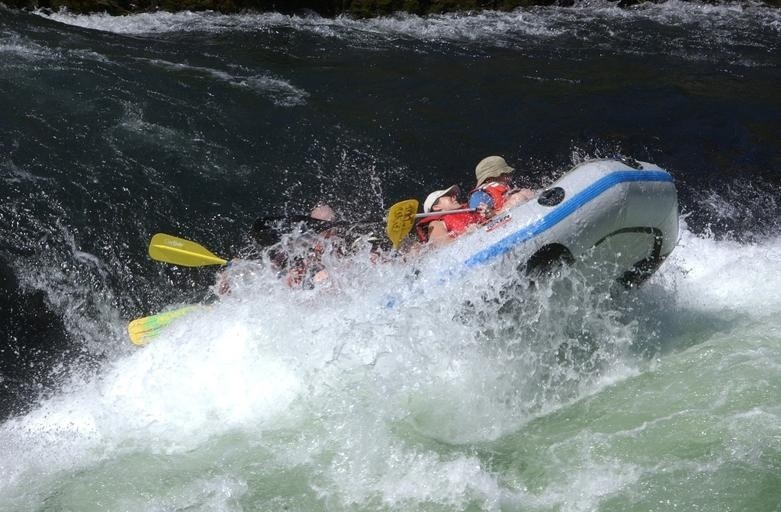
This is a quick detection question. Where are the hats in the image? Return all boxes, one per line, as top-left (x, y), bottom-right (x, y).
top-left (423, 186), bottom-right (460, 212)
top-left (474, 156), bottom-right (514, 188)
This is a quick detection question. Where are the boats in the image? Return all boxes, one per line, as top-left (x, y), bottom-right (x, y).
top-left (377, 154), bottom-right (679, 319)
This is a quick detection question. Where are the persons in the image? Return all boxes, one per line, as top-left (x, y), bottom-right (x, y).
top-left (272, 204), bottom-right (341, 288)
top-left (467, 156), bottom-right (534, 217)
top-left (416, 186), bottom-right (495, 244)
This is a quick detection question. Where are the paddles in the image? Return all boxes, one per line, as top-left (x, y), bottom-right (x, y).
top-left (386, 199), bottom-right (419, 261)
top-left (128, 305), bottom-right (214, 345)
top-left (148, 233), bottom-right (235, 267)
top-left (253, 207), bottom-right (481, 247)
